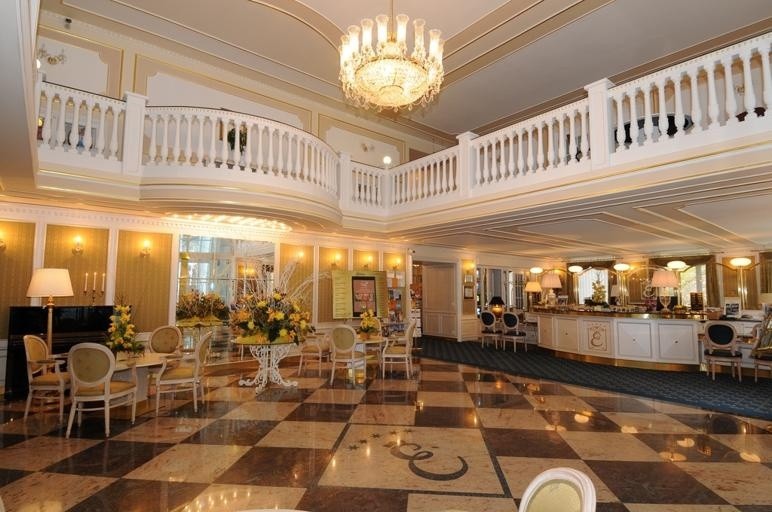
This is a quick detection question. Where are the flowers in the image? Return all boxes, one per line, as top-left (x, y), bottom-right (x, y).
top-left (360, 309), bottom-right (378, 332)
top-left (105, 293), bottom-right (148, 356)
top-left (175, 289), bottom-right (229, 320)
top-left (227, 288), bottom-right (316, 346)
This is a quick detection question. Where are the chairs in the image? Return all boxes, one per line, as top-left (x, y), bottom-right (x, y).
top-left (502, 312), bottom-right (527, 353)
top-left (297, 318), bottom-right (417, 386)
top-left (481, 311), bottom-right (503, 350)
top-left (23, 326), bottom-right (213, 440)
top-left (519, 467), bottom-right (597, 512)
top-left (753, 324), bottom-right (772, 382)
top-left (703, 319), bottom-right (742, 382)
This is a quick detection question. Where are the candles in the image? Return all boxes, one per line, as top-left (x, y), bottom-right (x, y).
top-left (93, 272), bottom-right (96, 290)
top-left (85, 272), bottom-right (88, 290)
top-left (102, 273), bottom-right (105, 291)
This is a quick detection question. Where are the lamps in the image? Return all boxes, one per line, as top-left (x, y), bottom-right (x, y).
top-left (524, 281), bottom-right (542, 305)
top-left (0, 233), bottom-right (6, 251)
top-left (651, 271), bottom-right (679, 314)
top-left (338, 0), bottom-right (445, 113)
top-left (489, 296), bottom-right (505, 319)
top-left (72, 236), bottom-right (83, 256)
top-left (542, 273), bottom-right (562, 305)
top-left (383, 156), bottom-right (391, 169)
top-left (25, 268), bottom-right (74, 355)
top-left (140, 242), bottom-right (151, 257)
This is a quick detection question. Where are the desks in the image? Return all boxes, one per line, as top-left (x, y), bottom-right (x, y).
top-left (614, 112), bottom-right (694, 144)
top-left (231, 338), bottom-right (306, 394)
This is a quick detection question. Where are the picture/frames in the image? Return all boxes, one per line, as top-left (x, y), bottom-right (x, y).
top-left (352, 277), bottom-right (376, 317)
top-left (463, 286), bottom-right (475, 300)
top-left (724, 301), bottom-right (741, 318)
top-left (220, 108), bottom-right (247, 146)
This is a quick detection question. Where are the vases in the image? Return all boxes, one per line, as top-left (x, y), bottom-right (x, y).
top-left (236, 335), bottom-right (289, 344)
top-left (114, 351), bottom-right (128, 361)
top-left (177, 315), bottom-right (223, 325)
